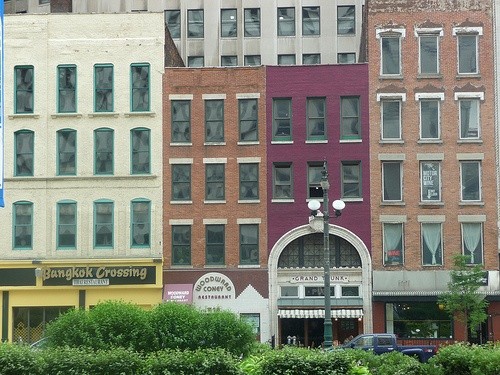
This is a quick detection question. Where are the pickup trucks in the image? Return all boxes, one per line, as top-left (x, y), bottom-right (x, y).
top-left (335, 333), bottom-right (436, 366)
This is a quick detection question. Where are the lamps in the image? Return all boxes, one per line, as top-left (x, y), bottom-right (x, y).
top-left (399, 304), bottom-right (410, 311)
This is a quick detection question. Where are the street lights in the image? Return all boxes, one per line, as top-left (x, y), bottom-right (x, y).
top-left (309, 161), bottom-right (346, 348)
